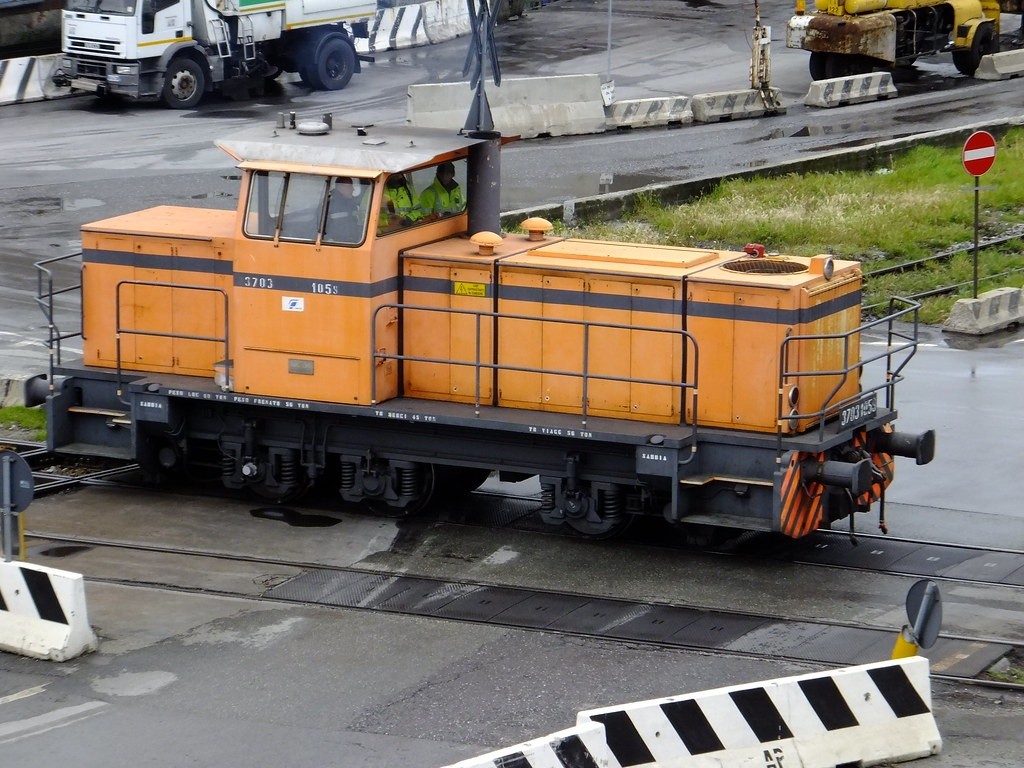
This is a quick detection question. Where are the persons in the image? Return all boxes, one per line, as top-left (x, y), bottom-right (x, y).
top-left (420, 161), bottom-right (465, 221)
top-left (316, 176), bottom-right (364, 243)
top-left (362, 173), bottom-right (422, 236)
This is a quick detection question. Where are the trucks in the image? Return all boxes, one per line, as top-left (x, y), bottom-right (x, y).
top-left (786, 0), bottom-right (1003, 82)
top-left (50, 0), bottom-right (379, 110)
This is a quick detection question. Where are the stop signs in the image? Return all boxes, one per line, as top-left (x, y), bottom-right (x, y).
top-left (961, 131), bottom-right (998, 176)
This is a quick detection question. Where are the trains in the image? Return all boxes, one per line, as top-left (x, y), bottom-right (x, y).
top-left (29, 108), bottom-right (935, 555)
